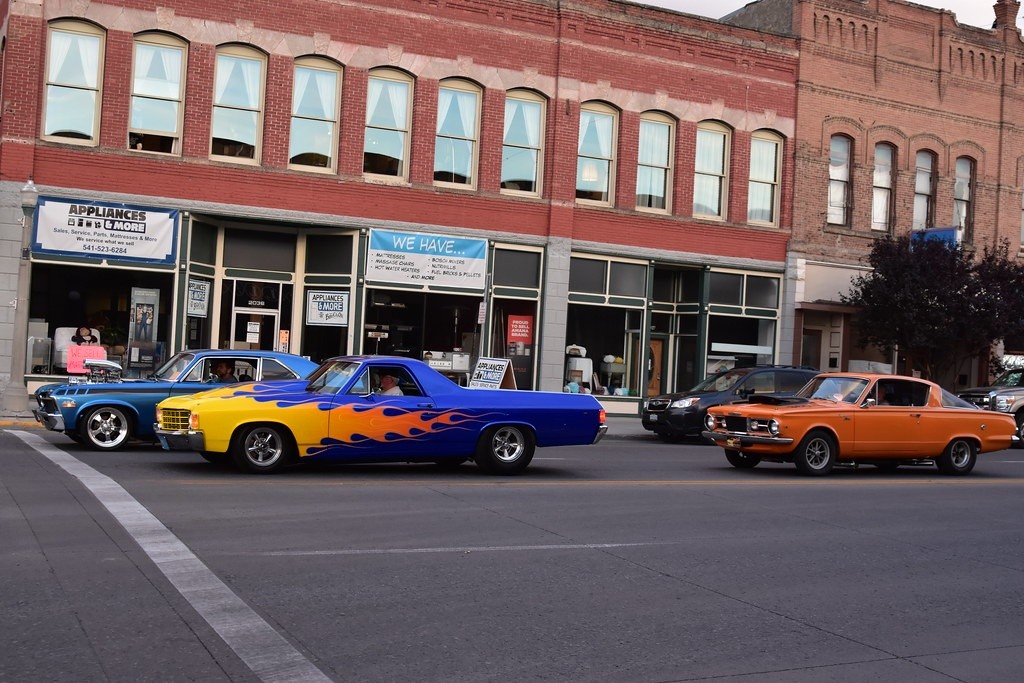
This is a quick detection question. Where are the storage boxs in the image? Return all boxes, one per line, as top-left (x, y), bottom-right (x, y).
top-left (423, 352), bottom-right (470, 371)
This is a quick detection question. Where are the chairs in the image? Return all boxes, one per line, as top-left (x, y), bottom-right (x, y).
top-left (239, 375), bottom-right (253, 382)
top-left (50, 328), bottom-right (107, 375)
top-left (885, 393), bottom-right (901, 405)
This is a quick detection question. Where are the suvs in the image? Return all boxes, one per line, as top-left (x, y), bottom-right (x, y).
top-left (641, 363), bottom-right (837, 443)
top-left (951, 368), bottom-right (1024, 449)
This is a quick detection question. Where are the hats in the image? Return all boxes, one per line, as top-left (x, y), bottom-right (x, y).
top-left (377, 368), bottom-right (399, 378)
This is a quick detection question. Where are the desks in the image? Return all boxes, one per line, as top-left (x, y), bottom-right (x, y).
top-left (437, 369), bottom-right (471, 387)
top-left (600, 362), bottom-right (627, 388)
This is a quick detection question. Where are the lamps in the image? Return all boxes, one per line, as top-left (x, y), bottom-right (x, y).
top-left (581, 160), bottom-right (597, 198)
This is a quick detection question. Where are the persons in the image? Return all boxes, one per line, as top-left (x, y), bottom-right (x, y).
top-left (370, 370), bottom-right (404, 396)
top-left (866, 383), bottom-right (890, 406)
top-left (207, 360), bottom-right (239, 384)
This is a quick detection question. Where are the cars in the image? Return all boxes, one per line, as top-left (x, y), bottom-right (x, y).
top-left (31, 347), bottom-right (364, 451)
top-left (701, 372), bottom-right (1017, 477)
top-left (153, 354), bottom-right (608, 477)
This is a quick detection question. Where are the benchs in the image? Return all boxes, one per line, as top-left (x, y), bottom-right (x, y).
top-left (401, 386), bottom-right (419, 396)
